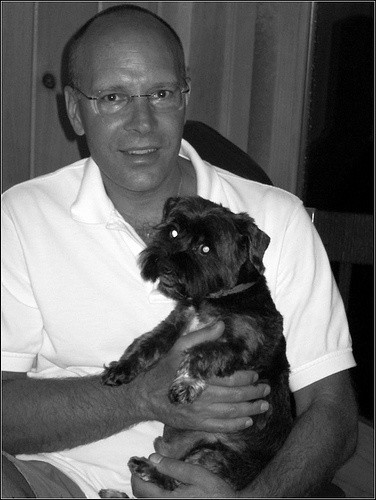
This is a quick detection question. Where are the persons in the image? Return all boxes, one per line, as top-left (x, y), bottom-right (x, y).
top-left (1, 4), bottom-right (357, 499)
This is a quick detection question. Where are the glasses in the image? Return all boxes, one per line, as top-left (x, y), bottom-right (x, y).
top-left (76, 87), bottom-right (190, 115)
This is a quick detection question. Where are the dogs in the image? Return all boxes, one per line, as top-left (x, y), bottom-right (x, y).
top-left (98, 196), bottom-right (296, 498)
